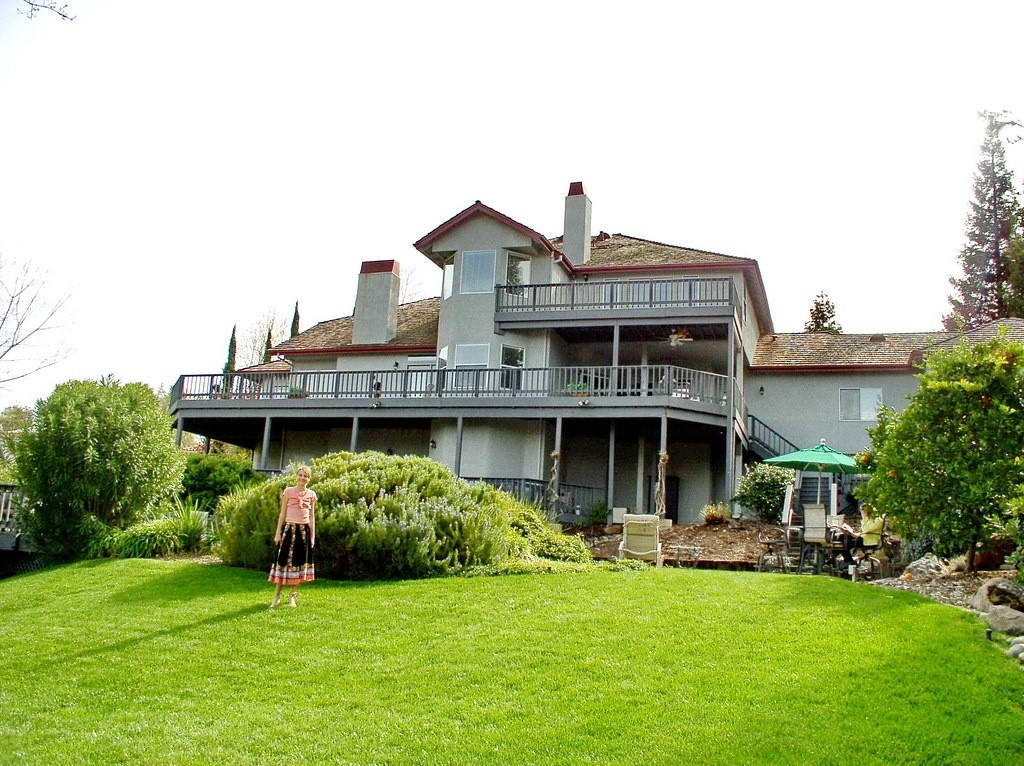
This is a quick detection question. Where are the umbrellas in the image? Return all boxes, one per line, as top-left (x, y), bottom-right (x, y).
top-left (762, 438), bottom-right (865, 564)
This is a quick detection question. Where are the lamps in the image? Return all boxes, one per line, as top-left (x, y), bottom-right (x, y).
top-left (429, 438), bottom-right (436, 449)
top-left (393, 361), bottom-right (399, 370)
top-left (669, 340), bottom-right (677, 347)
top-left (759, 385), bottom-right (764, 395)
top-left (386, 448), bottom-right (394, 456)
top-left (584, 273), bottom-right (589, 280)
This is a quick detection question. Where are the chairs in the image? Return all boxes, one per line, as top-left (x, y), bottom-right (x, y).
top-left (619, 513), bottom-right (664, 567)
top-left (757, 503), bottom-right (889, 582)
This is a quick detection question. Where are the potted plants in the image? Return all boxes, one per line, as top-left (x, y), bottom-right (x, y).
top-left (288, 387), bottom-right (309, 399)
top-left (563, 383), bottom-right (592, 397)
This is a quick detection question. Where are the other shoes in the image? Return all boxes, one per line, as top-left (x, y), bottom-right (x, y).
top-left (270, 593), bottom-right (281, 608)
top-left (288, 592), bottom-right (297, 607)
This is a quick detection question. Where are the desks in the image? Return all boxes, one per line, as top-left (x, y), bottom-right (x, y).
top-left (786, 526), bottom-right (847, 575)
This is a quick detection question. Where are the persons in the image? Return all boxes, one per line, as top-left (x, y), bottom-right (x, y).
top-left (841, 503), bottom-right (884, 570)
top-left (268, 466), bottom-right (318, 607)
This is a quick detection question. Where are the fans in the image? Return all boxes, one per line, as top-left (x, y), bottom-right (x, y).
top-left (654, 326), bottom-right (693, 346)
